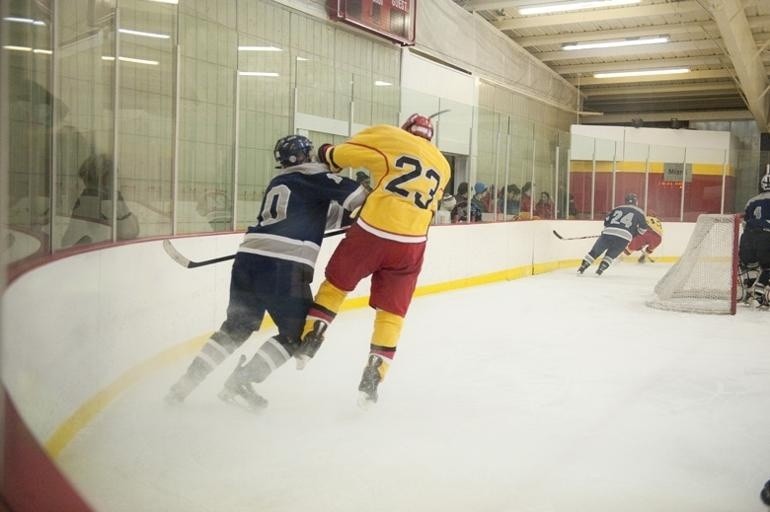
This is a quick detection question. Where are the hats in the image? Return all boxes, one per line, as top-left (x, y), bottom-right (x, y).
top-left (474, 182), bottom-right (487, 194)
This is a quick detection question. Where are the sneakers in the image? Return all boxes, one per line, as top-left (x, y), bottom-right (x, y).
top-left (293, 330), bottom-right (324, 358)
top-left (579, 261), bottom-right (590, 272)
top-left (359, 367), bottom-right (381, 402)
top-left (597, 266), bottom-right (605, 274)
top-left (224, 367), bottom-right (267, 406)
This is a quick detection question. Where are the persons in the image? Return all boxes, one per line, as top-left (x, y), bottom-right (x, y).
top-left (340, 171), bottom-right (373, 233)
top-left (292, 116), bottom-right (451, 403)
top-left (436, 180), bottom-right (556, 224)
top-left (738, 173), bottom-right (770, 305)
top-left (166, 133), bottom-right (369, 411)
top-left (61, 152), bottom-right (140, 249)
top-left (616, 209), bottom-right (663, 264)
top-left (577, 193), bottom-right (646, 276)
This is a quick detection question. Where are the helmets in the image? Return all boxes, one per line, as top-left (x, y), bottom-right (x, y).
top-left (274, 135), bottom-right (312, 169)
top-left (402, 114), bottom-right (433, 141)
top-left (625, 193), bottom-right (638, 206)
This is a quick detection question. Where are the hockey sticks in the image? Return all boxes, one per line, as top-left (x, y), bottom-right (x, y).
top-left (553, 230), bottom-right (602, 240)
top-left (162, 228), bottom-right (346, 268)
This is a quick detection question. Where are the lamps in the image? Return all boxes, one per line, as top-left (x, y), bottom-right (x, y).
top-left (561, 33), bottom-right (671, 51)
top-left (593, 66), bottom-right (690, 78)
top-left (518, 0), bottom-right (642, 15)
